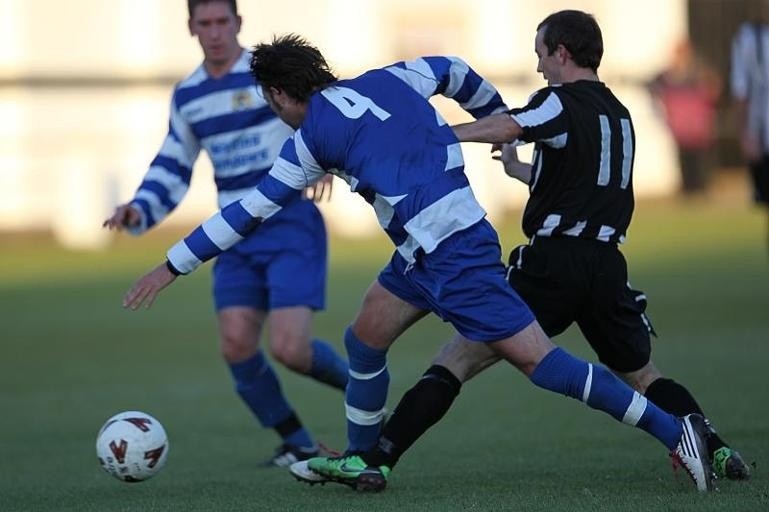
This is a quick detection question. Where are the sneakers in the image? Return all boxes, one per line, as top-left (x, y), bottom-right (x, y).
top-left (255, 442), bottom-right (324, 468)
top-left (674, 416), bottom-right (717, 493)
top-left (307, 453), bottom-right (392, 494)
top-left (704, 420), bottom-right (753, 482)
top-left (288, 458), bottom-right (336, 485)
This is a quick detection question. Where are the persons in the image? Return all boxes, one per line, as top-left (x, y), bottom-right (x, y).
top-left (303, 11), bottom-right (751, 492)
top-left (120, 32), bottom-right (721, 495)
top-left (650, 32), bottom-right (736, 202)
top-left (98, 2), bottom-right (353, 474)
top-left (728, 1), bottom-right (768, 206)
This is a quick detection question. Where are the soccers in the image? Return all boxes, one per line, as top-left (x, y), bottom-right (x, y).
top-left (95, 410), bottom-right (169, 484)
